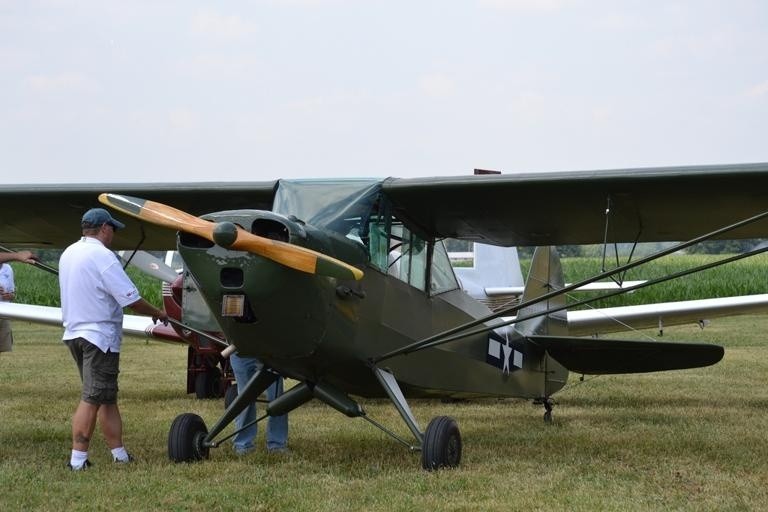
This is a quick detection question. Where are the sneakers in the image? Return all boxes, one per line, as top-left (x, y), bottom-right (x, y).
top-left (114, 453), bottom-right (135, 463)
top-left (66, 459), bottom-right (90, 472)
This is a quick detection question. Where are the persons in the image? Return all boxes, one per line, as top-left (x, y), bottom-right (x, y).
top-left (229, 351), bottom-right (287, 455)
top-left (0, 250), bottom-right (39, 352)
top-left (58, 208), bottom-right (168, 471)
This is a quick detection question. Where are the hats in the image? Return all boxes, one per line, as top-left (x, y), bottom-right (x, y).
top-left (81, 207), bottom-right (126, 228)
